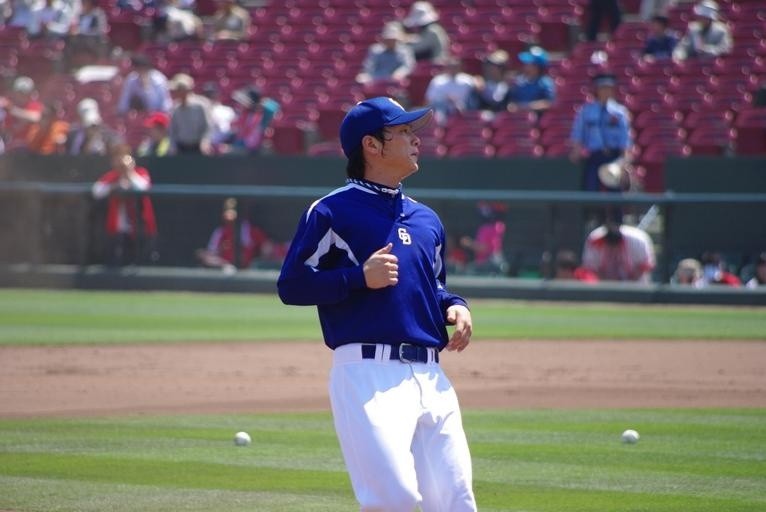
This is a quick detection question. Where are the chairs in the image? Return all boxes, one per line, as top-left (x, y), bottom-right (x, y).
top-left (1, 0), bottom-right (765, 156)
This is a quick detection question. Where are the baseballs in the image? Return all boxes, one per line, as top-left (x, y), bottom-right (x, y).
top-left (236, 431), bottom-right (250, 444)
top-left (623, 430), bottom-right (638, 443)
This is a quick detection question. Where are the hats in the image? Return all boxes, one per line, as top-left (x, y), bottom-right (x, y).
top-left (143, 112), bottom-right (168, 128)
top-left (516, 45), bottom-right (550, 65)
top-left (74, 97), bottom-right (103, 128)
top-left (590, 75), bottom-right (616, 88)
top-left (167, 73), bottom-right (196, 91)
top-left (372, 21), bottom-right (405, 44)
top-left (692, 0), bottom-right (720, 22)
top-left (339, 96), bottom-right (434, 155)
top-left (402, 1), bottom-right (442, 29)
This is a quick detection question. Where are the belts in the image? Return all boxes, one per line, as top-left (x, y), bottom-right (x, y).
top-left (362, 344), bottom-right (439, 365)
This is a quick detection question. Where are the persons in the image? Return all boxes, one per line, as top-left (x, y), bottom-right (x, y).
top-left (277, 97), bottom-right (477, 511)
